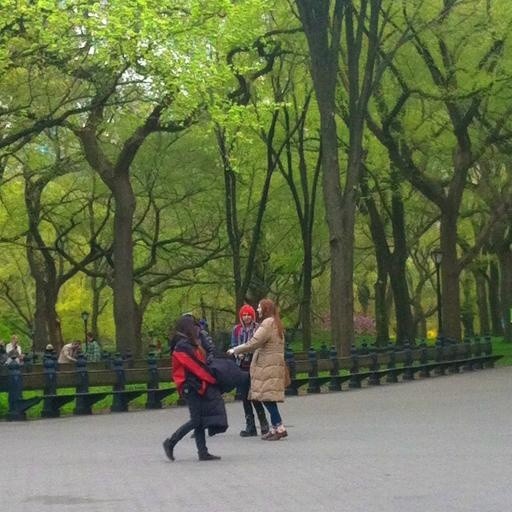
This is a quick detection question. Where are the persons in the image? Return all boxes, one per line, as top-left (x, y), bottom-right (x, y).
top-left (81, 331), bottom-right (102, 363)
top-left (57, 339), bottom-right (81, 364)
top-left (190, 316), bottom-right (219, 396)
top-left (4, 335), bottom-right (22, 365)
top-left (231, 303), bottom-right (271, 440)
top-left (162, 315), bottom-right (222, 461)
top-left (224, 298), bottom-right (288, 441)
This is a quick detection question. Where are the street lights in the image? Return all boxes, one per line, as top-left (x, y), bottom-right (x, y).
top-left (81, 309), bottom-right (93, 354)
top-left (430, 245), bottom-right (450, 347)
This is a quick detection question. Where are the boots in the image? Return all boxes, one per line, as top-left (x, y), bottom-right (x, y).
top-left (257, 411), bottom-right (269, 435)
top-left (240, 413), bottom-right (258, 437)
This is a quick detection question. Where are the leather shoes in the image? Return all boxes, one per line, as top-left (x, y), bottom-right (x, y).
top-left (262, 432), bottom-right (272, 440)
top-left (268, 431), bottom-right (288, 441)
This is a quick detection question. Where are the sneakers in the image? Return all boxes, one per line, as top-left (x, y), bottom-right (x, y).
top-left (163, 438), bottom-right (175, 461)
top-left (198, 452), bottom-right (222, 461)
